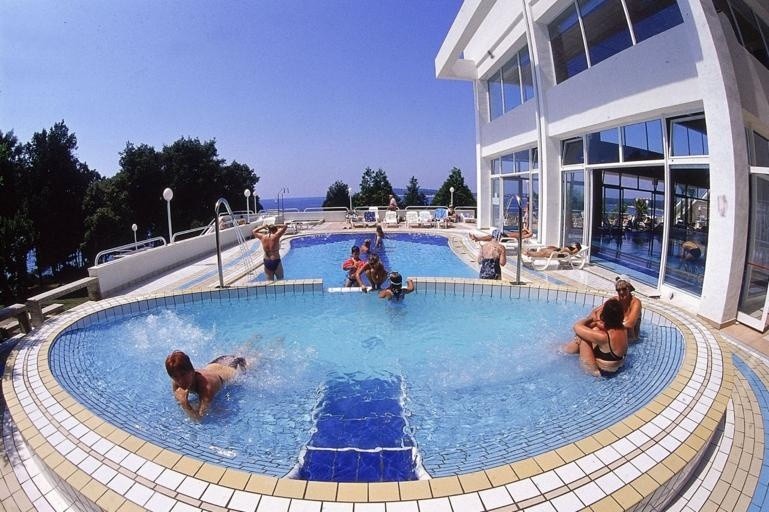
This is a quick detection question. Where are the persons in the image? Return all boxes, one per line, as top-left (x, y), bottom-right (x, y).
top-left (591, 273), bottom-right (643, 342)
top-left (237, 214), bottom-right (246, 225)
top-left (359, 239), bottom-right (371, 252)
top-left (386, 194), bottom-right (396, 211)
top-left (378, 271), bottom-right (414, 304)
top-left (342, 246), bottom-right (365, 287)
top-left (219, 218), bottom-right (229, 230)
top-left (478, 229), bottom-right (507, 281)
top-left (354, 252), bottom-right (388, 293)
top-left (524, 240), bottom-right (581, 258)
top-left (374, 225), bottom-right (384, 250)
top-left (251, 223), bottom-right (288, 281)
top-left (673, 240), bottom-right (702, 273)
top-left (469, 227), bottom-right (533, 242)
top-left (561, 296), bottom-right (628, 380)
top-left (164, 331), bottom-right (284, 422)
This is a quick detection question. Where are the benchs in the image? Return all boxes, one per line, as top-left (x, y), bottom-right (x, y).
top-left (0, 276), bottom-right (101, 339)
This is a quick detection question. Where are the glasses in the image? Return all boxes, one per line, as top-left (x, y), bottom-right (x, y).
top-left (616, 288), bottom-right (628, 292)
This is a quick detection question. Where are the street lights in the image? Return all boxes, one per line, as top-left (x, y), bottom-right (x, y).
top-left (163, 187), bottom-right (174, 243)
top-left (348, 187), bottom-right (353, 210)
top-left (244, 188), bottom-right (251, 224)
top-left (449, 186), bottom-right (455, 205)
top-left (131, 223), bottom-right (138, 251)
top-left (253, 191), bottom-right (257, 214)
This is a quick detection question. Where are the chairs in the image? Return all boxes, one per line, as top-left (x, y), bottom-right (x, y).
top-left (520, 244), bottom-right (590, 271)
top-left (351, 206), bottom-right (449, 228)
top-left (461, 213), bottom-right (474, 223)
top-left (468, 233), bottom-right (537, 250)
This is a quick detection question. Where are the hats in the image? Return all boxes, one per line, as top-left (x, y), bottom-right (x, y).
top-left (614, 276), bottom-right (635, 290)
